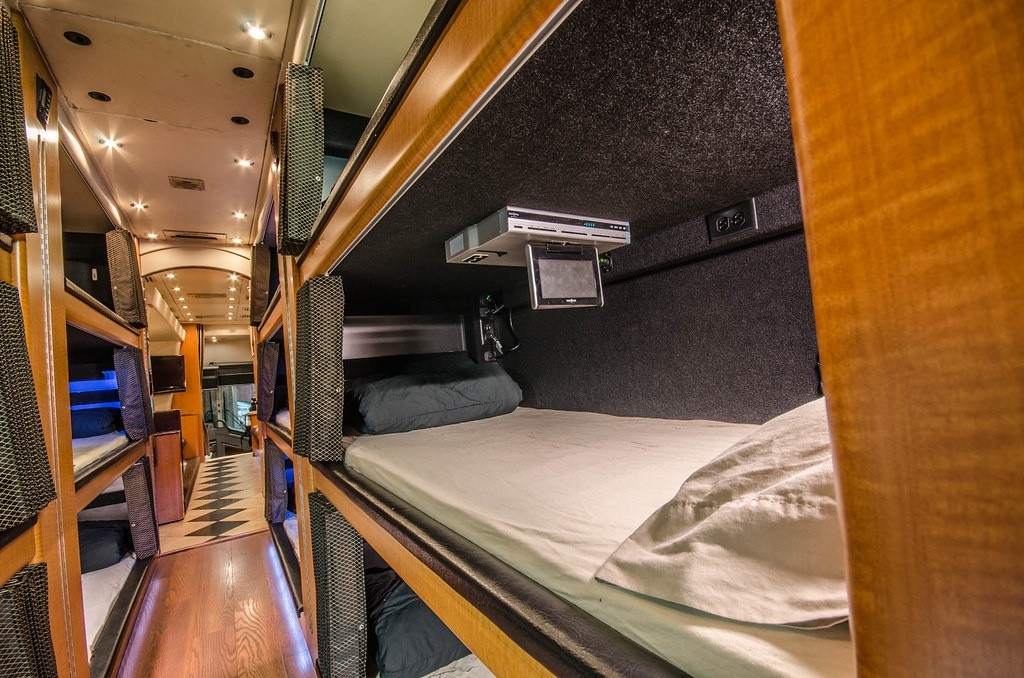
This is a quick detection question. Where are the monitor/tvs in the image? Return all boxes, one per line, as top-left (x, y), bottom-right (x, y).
top-left (150, 354), bottom-right (185, 394)
top-left (525, 242), bottom-right (605, 310)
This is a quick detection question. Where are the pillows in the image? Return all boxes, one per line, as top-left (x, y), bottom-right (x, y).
top-left (593, 396), bottom-right (848, 631)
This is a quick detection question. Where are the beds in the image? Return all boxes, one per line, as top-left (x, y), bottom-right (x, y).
top-left (248, 1), bottom-right (1024, 677)
top-left (0, 0), bottom-right (206, 678)
